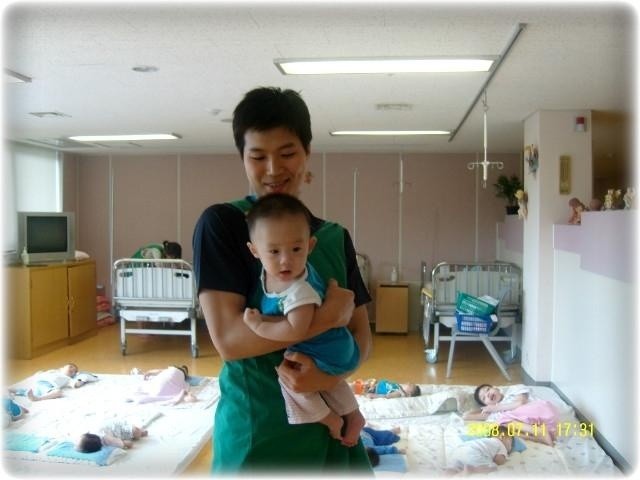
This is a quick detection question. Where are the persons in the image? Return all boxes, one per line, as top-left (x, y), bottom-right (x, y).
top-left (5, 387), bottom-right (26, 429)
top-left (347, 377), bottom-right (421, 400)
top-left (143, 364), bottom-right (188, 380)
top-left (360, 422), bottom-right (407, 467)
top-left (74, 417), bottom-right (147, 452)
top-left (25, 363), bottom-right (89, 401)
top-left (242, 191), bottom-right (366, 447)
top-left (463, 384), bottom-right (561, 447)
top-left (442, 425), bottom-right (513, 477)
top-left (125, 240), bottom-right (182, 278)
top-left (192, 87), bottom-right (371, 475)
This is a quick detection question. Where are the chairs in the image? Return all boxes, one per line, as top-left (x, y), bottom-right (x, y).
top-left (445, 287), bottom-right (511, 381)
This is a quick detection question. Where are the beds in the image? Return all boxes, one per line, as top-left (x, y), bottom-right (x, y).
top-left (418, 255), bottom-right (521, 362)
top-left (111, 256), bottom-right (207, 360)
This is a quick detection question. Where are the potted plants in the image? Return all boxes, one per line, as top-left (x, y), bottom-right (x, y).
top-left (492, 174), bottom-right (524, 214)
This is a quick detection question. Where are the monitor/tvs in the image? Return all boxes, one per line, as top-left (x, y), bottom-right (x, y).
top-left (17, 211), bottom-right (75, 262)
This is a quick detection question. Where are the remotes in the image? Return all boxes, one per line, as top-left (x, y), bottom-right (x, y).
top-left (27, 264), bottom-right (48, 267)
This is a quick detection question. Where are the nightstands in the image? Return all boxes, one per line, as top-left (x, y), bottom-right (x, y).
top-left (374, 280), bottom-right (411, 336)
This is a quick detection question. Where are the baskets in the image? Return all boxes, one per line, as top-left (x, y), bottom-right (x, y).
top-left (455, 290), bottom-right (497, 333)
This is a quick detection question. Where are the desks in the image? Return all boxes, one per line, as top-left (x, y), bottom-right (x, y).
top-left (5, 258), bottom-right (99, 362)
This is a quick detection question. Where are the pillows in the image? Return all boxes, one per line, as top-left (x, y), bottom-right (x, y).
top-left (73, 371), bottom-right (102, 385)
top-left (452, 426), bottom-right (526, 453)
top-left (41, 438), bottom-right (125, 466)
top-left (186, 375), bottom-right (211, 389)
top-left (4, 432), bottom-right (47, 462)
